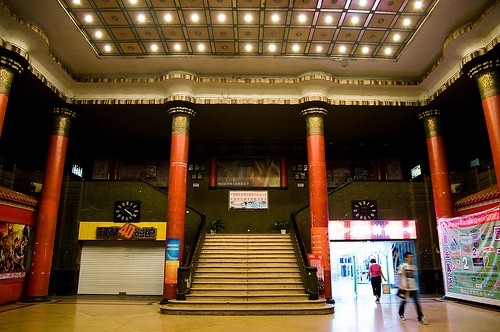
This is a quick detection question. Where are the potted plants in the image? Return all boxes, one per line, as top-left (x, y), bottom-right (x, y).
top-left (272, 216), bottom-right (289, 235)
top-left (208, 215), bottom-right (225, 235)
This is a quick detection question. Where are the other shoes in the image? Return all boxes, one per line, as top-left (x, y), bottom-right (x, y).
top-left (418, 318), bottom-right (428, 325)
top-left (374, 298), bottom-right (380, 303)
top-left (399, 315), bottom-right (407, 320)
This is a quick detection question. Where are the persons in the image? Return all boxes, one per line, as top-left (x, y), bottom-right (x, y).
top-left (366, 259), bottom-right (387, 303)
top-left (397, 252), bottom-right (428, 325)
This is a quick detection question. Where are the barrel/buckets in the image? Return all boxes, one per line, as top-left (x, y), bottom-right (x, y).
top-left (382, 280), bottom-right (390, 294)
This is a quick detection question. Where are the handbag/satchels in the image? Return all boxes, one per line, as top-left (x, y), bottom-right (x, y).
top-left (382, 280), bottom-right (390, 294)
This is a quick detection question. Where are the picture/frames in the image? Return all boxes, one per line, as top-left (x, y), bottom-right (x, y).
top-left (188, 161), bottom-right (205, 189)
top-left (290, 163), bottom-right (309, 188)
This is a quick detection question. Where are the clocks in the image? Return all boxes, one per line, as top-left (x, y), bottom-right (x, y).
top-left (113, 200), bottom-right (140, 222)
top-left (351, 200), bottom-right (378, 221)
